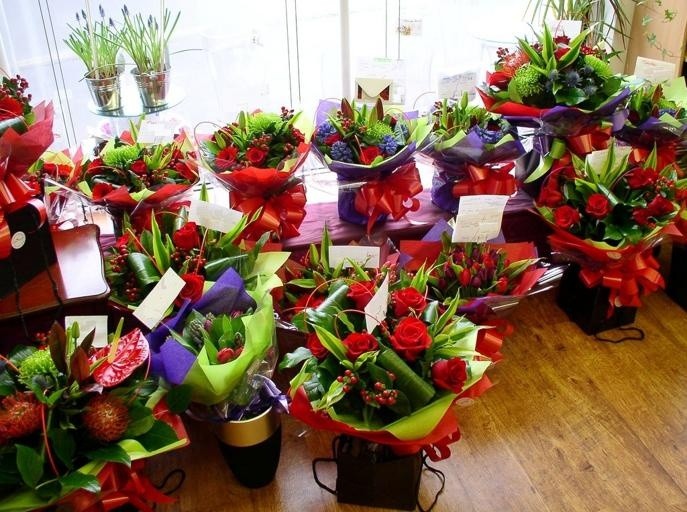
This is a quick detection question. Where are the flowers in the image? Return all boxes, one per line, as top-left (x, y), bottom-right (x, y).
top-left (308, 87), bottom-right (428, 228)
top-left (108, 227), bottom-right (286, 330)
top-left (474, 20), bottom-right (633, 120)
top-left (396, 219), bottom-right (547, 324)
top-left (621, 72), bottom-right (687, 146)
top-left (286, 238), bottom-right (498, 511)
top-left (169, 305), bottom-right (287, 417)
top-left (2, 315), bottom-right (189, 511)
top-left (411, 94), bottom-right (524, 207)
top-left (1, 68), bottom-right (56, 219)
top-left (83, 121), bottom-right (203, 226)
top-left (194, 102), bottom-right (312, 242)
top-left (537, 142), bottom-right (686, 340)
top-left (28, 147), bottom-right (80, 225)
